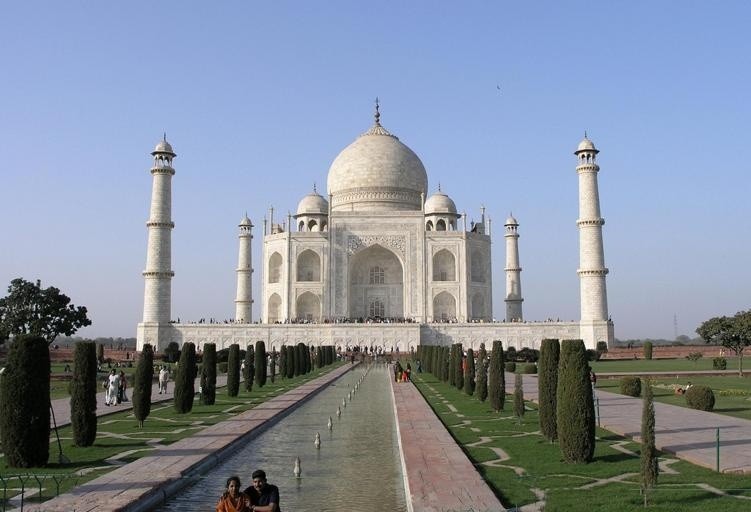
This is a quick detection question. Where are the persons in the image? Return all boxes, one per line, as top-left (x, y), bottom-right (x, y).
top-left (339, 343), bottom-right (387, 364)
top-left (104, 368), bottom-right (120, 405)
top-left (240, 358), bottom-right (246, 378)
top-left (177, 316), bottom-right (181, 323)
top-left (221, 468), bottom-right (283, 512)
top-left (397, 369), bottom-right (402, 383)
top-left (119, 369), bottom-right (129, 401)
top-left (403, 370), bottom-right (407, 383)
top-left (406, 361), bottom-right (411, 380)
top-left (684, 381), bottom-right (694, 394)
top-left (157, 365), bottom-right (170, 394)
top-left (214, 475), bottom-right (250, 511)
top-left (589, 364), bottom-right (598, 401)
top-left (417, 358), bottom-right (423, 373)
top-left (394, 360), bottom-right (402, 381)
top-left (199, 315), bottom-right (525, 323)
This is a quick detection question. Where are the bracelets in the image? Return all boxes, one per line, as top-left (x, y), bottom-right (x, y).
top-left (250, 503), bottom-right (256, 510)
